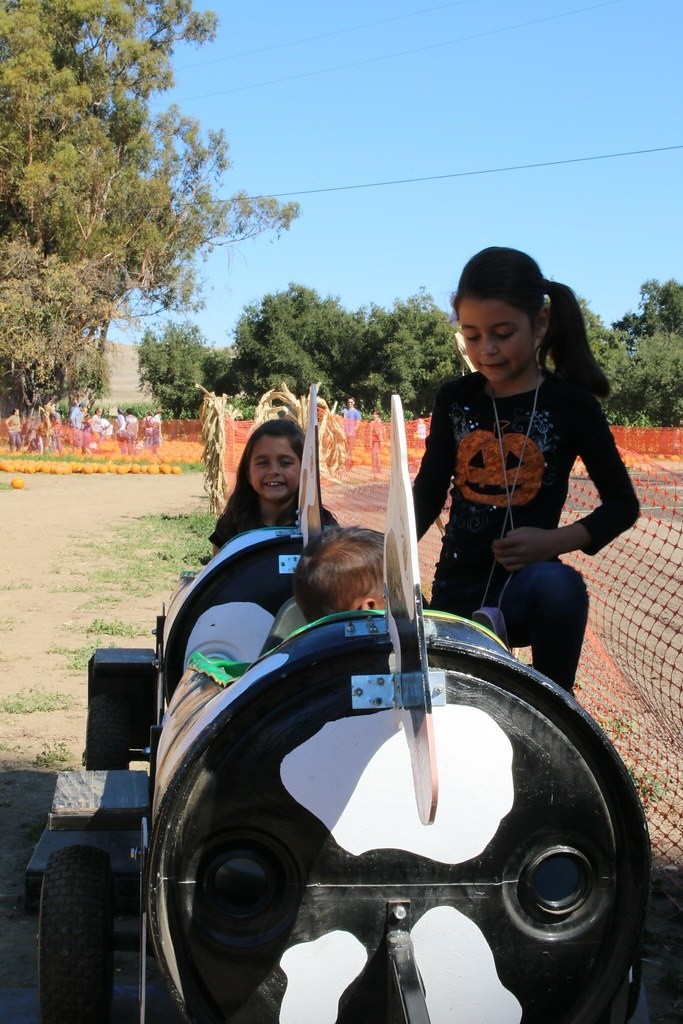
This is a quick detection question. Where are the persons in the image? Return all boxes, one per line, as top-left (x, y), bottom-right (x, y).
top-left (368, 409), bottom-right (386, 475)
top-left (207, 417), bottom-right (342, 559)
top-left (413, 417), bottom-right (426, 451)
top-left (408, 243), bottom-right (639, 701)
top-left (292, 525), bottom-right (388, 625)
top-left (340, 396), bottom-right (362, 472)
top-left (4, 400), bottom-right (164, 458)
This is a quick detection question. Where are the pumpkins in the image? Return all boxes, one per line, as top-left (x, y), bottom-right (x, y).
top-left (11, 477), bottom-right (25, 489)
top-left (0, 441), bottom-right (205, 475)
top-left (350, 442), bottom-right (425, 467)
